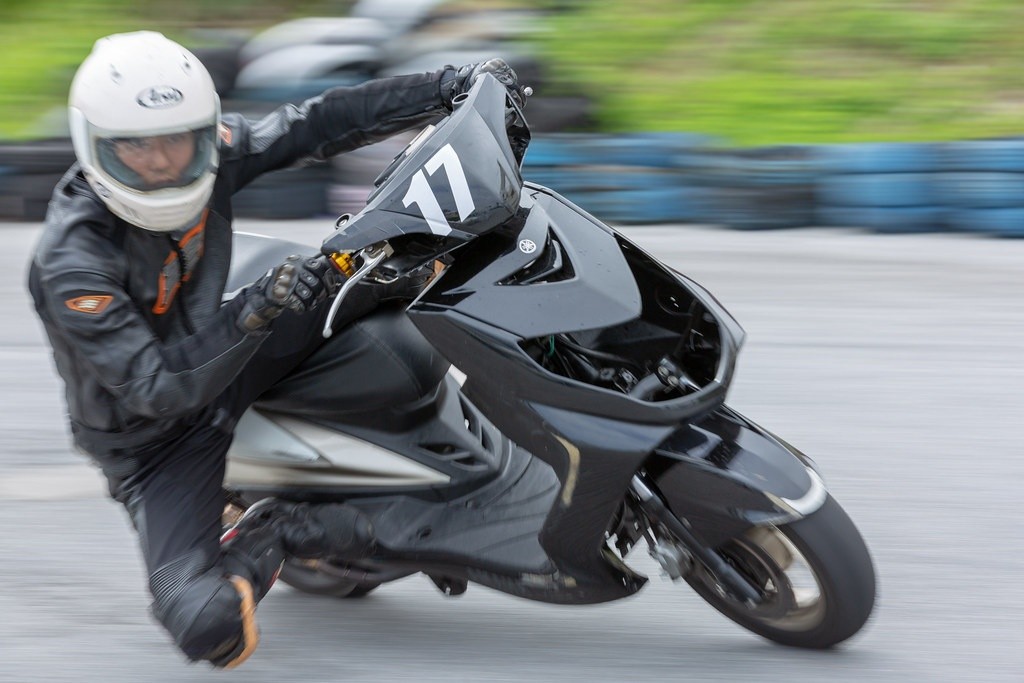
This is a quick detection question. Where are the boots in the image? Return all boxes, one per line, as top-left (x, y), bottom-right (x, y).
top-left (219, 496), bottom-right (377, 600)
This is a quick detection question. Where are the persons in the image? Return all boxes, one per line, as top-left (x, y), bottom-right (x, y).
top-left (27, 31), bottom-right (527, 671)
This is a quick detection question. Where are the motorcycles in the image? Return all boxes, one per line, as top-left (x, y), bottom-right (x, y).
top-left (213, 64), bottom-right (880, 652)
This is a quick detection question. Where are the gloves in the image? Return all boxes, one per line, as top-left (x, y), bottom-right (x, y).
top-left (440, 57), bottom-right (528, 129)
top-left (223, 255), bottom-right (338, 336)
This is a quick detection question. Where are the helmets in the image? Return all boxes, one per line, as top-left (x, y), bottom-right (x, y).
top-left (68, 29), bottom-right (221, 231)
